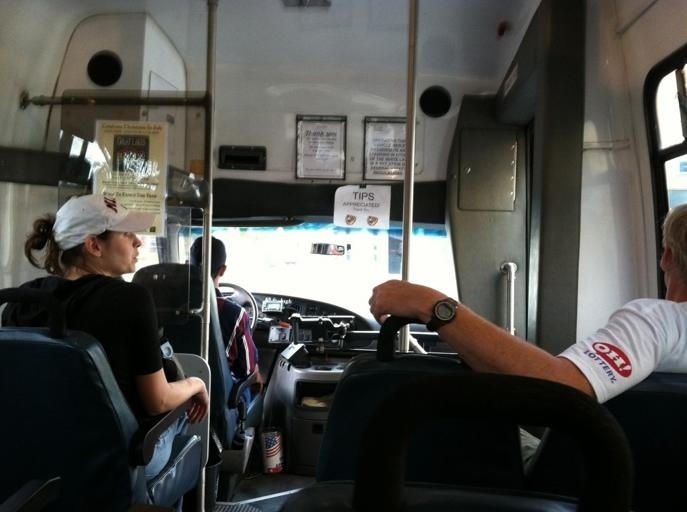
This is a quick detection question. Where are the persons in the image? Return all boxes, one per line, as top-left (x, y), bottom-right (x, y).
top-left (2, 194), bottom-right (209, 485)
top-left (368, 205), bottom-right (686, 483)
top-left (183, 234), bottom-right (265, 413)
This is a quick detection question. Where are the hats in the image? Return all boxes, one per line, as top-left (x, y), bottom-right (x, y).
top-left (52, 192), bottom-right (155, 252)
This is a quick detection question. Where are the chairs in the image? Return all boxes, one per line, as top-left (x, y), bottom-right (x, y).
top-left (131, 262), bottom-right (267, 502)
top-left (1, 326), bottom-right (206, 511)
top-left (316, 355), bottom-right (687, 512)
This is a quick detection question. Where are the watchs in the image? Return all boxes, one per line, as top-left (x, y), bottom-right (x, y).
top-left (425, 297), bottom-right (459, 333)
